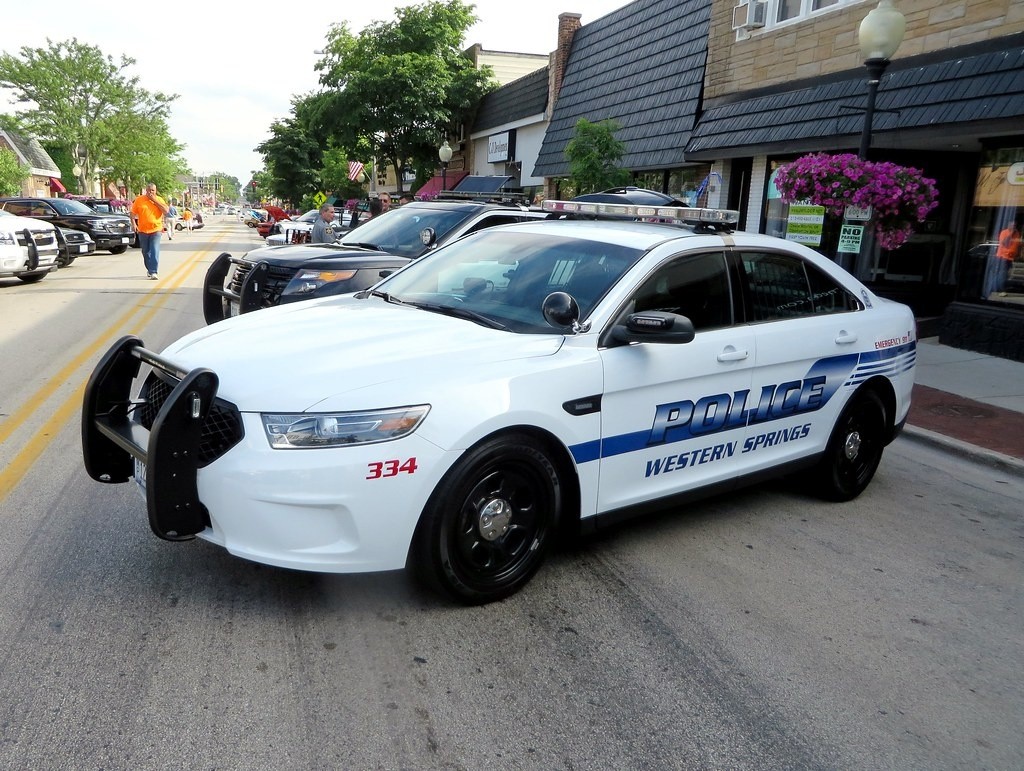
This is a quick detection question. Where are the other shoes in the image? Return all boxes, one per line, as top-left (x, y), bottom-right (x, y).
top-left (168, 237), bottom-right (171, 240)
top-left (999, 292), bottom-right (1008, 297)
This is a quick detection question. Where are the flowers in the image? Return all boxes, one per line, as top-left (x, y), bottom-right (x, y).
top-left (772, 153), bottom-right (937, 249)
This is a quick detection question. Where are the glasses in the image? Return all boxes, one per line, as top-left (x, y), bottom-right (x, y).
top-left (379, 199), bottom-right (389, 204)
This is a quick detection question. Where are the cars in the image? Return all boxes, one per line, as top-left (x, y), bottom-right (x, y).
top-left (241, 208), bottom-right (373, 248)
top-left (82, 200), bottom-right (919, 607)
top-left (174, 215), bottom-right (204, 231)
top-left (228, 208), bottom-right (236, 215)
top-left (214, 204), bottom-right (226, 216)
top-left (1, 210), bottom-right (97, 287)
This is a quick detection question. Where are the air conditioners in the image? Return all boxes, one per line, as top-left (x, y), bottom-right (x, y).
top-left (732, 0), bottom-right (768, 30)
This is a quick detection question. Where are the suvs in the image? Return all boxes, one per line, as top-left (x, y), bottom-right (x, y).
top-left (0, 197), bottom-right (137, 255)
top-left (201, 187), bottom-right (691, 327)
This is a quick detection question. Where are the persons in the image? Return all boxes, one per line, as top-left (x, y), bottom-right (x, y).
top-left (992, 220), bottom-right (1020, 296)
top-left (131, 183), bottom-right (169, 280)
top-left (370, 192), bottom-right (390, 216)
top-left (165, 201), bottom-right (178, 240)
top-left (311, 203), bottom-right (338, 242)
top-left (183, 208), bottom-right (192, 234)
top-left (399, 194), bottom-right (414, 205)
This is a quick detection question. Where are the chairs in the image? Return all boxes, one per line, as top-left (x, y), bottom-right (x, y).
top-left (563, 261), bottom-right (609, 318)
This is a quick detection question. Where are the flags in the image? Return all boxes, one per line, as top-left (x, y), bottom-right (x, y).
top-left (348, 161), bottom-right (363, 180)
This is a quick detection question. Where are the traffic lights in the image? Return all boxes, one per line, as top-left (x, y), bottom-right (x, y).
top-left (253, 182), bottom-right (256, 192)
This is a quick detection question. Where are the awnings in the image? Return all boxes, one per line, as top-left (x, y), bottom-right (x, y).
top-left (415, 171), bottom-right (468, 196)
top-left (50, 177), bottom-right (66, 192)
top-left (454, 175), bottom-right (509, 192)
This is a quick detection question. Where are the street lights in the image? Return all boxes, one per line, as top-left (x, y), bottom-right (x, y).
top-left (117, 178), bottom-right (123, 199)
top-left (357, 171), bottom-right (366, 203)
top-left (858, 3), bottom-right (906, 169)
top-left (439, 140), bottom-right (453, 191)
top-left (72, 164), bottom-right (83, 196)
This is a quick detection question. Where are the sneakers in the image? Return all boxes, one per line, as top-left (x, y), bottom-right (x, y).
top-left (146, 270), bottom-right (159, 280)
